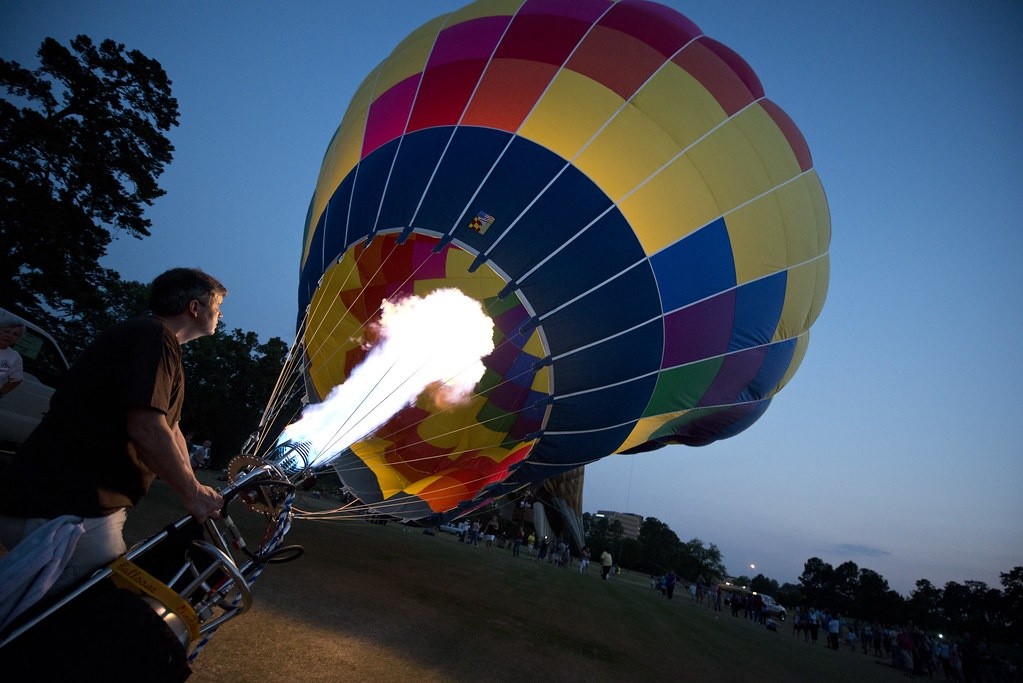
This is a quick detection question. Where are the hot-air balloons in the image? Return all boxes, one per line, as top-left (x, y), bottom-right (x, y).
top-left (0, 0), bottom-right (835, 681)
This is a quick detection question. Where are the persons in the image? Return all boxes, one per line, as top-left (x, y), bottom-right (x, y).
top-left (309, 486), bottom-right (440, 537)
top-left (0, 268), bottom-right (225, 596)
top-left (0, 316), bottom-right (24, 400)
top-left (455, 510), bottom-right (1023, 682)
top-left (189, 441), bottom-right (212, 474)
top-left (184, 431), bottom-right (193, 452)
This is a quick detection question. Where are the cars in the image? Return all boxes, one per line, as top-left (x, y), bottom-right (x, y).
top-left (0, 306), bottom-right (69, 444)
top-left (756, 593), bottom-right (788, 621)
top-left (437, 522), bottom-right (464, 536)
